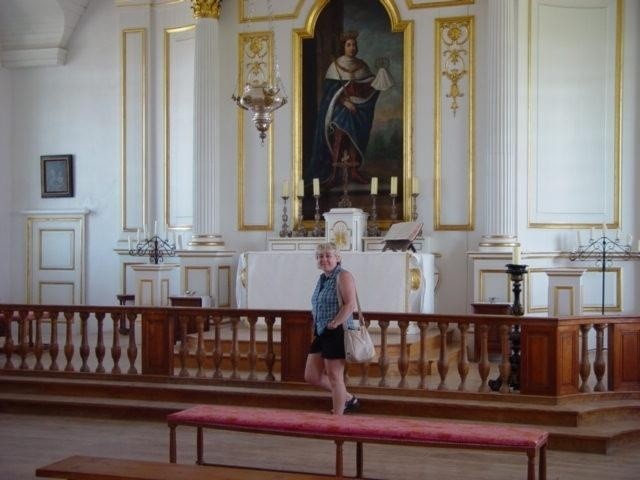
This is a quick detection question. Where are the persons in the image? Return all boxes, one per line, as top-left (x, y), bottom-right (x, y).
top-left (304, 242), bottom-right (359, 416)
top-left (304, 29), bottom-right (380, 189)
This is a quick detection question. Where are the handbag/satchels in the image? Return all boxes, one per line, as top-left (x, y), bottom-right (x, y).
top-left (344, 327), bottom-right (376, 365)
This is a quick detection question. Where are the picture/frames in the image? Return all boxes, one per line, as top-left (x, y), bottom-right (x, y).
top-left (40, 155), bottom-right (73, 198)
top-left (291, 0), bottom-right (413, 229)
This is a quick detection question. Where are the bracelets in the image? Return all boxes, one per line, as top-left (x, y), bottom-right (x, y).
top-left (331, 320), bottom-right (336, 323)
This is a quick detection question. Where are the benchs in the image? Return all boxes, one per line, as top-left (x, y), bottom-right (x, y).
top-left (168, 403), bottom-right (550, 479)
top-left (34, 451), bottom-right (336, 480)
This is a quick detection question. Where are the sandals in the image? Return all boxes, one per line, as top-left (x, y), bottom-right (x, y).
top-left (331, 395), bottom-right (359, 414)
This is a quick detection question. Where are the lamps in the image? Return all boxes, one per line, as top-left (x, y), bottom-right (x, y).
top-left (232, 1), bottom-right (288, 147)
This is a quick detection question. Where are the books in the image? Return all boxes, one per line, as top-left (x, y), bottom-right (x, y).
top-left (383, 221), bottom-right (422, 242)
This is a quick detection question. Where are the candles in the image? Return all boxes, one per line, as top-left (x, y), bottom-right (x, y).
top-left (296, 178), bottom-right (305, 195)
top-left (281, 178), bottom-right (290, 196)
top-left (313, 177), bottom-right (321, 195)
top-left (371, 177), bottom-right (379, 195)
top-left (512, 245), bottom-right (522, 264)
top-left (390, 176), bottom-right (398, 194)
top-left (127, 219), bottom-right (183, 253)
top-left (574, 219), bottom-right (635, 246)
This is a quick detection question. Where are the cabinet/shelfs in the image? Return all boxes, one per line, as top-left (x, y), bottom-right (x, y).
top-left (471, 302), bottom-right (511, 365)
top-left (170, 296), bottom-right (210, 344)
